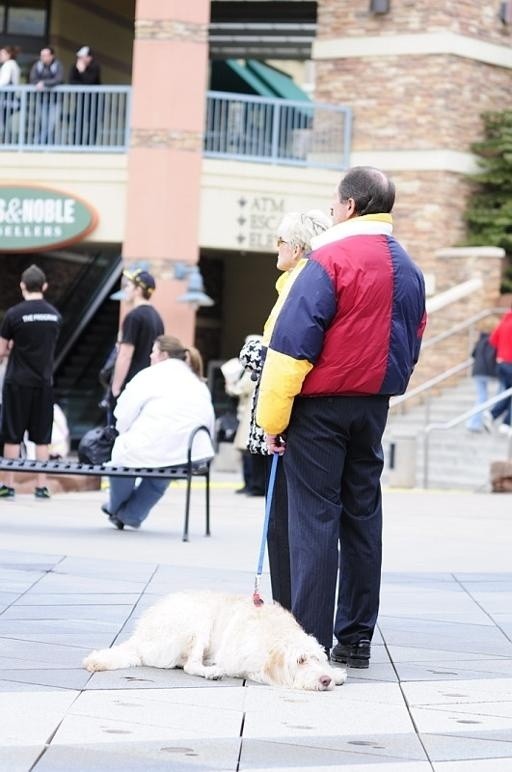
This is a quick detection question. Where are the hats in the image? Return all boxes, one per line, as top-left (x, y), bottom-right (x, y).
top-left (123, 267), bottom-right (156, 294)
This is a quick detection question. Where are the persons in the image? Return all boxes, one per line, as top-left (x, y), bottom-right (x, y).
top-left (30, 45), bottom-right (65, 146)
top-left (99, 336), bottom-right (217, 532)
top-left (110, 268), bottom-right (167, 437)
top-left (0, 45), bottom-right (27, 145)
top-left (470, 316), bottom-right (506, 435)
top-left (251, 165), bottom-right (427, 675)
top-left (238, 209), bottom-right (335, 617)
top-left (220, 334), bottom-right (273, 498)
top-left (0, 262), bottom-right (63, 503)
top-left (480, 300), bottom-right (512, 440)
top-left (70, 45), bottom-right (107, 146)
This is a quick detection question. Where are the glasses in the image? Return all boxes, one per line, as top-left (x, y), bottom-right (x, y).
top-left (277, 236), bottom-right (287, 247)
top-left (329, 200), bottom-right (340, 216)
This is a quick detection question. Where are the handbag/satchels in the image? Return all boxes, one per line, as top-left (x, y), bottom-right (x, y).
top-left (78, 425), bottom-right (119, 465)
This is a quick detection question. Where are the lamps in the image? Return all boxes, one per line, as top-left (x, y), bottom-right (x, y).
top-left (104, 255), bottom-right (214, 316)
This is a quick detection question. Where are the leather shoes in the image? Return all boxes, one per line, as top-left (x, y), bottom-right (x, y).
top-left (331, 645), bottom-right (370, 669)
top-left (103, 503), bottom-right (124, 531)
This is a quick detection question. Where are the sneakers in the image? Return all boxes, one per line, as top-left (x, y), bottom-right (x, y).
top-left (34, 487), bottom-right (51, 498)
top-left (0, 485), bottom-right (15, 499)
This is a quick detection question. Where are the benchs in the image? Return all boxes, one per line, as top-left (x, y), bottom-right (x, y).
top-left (0, 425), bottom-right (213, 542)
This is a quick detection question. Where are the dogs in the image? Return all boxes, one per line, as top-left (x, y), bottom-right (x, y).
top-left (82, 588), bottom-right (347, 692)
